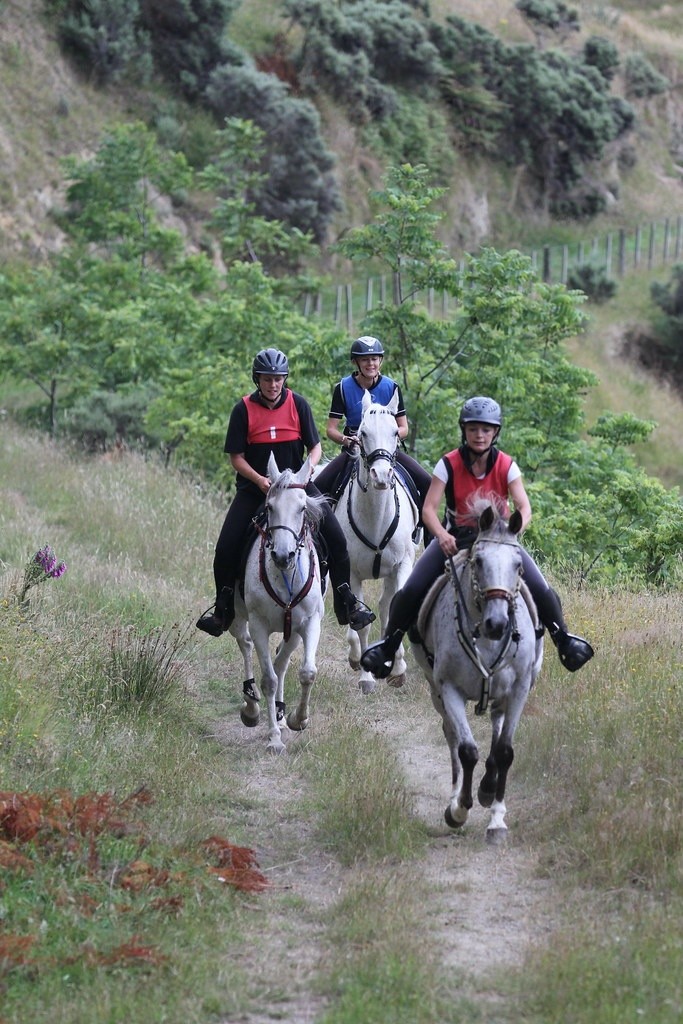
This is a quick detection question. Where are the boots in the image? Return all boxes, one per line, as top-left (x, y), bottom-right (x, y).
top-left (534, 587), bottom-right (595, 673)
top-left (195, 567), bottom-right (235, 638)
top-left (328, 556), bottom-right (377, 634)
top-left (359, 621), bottom-right (406, 680)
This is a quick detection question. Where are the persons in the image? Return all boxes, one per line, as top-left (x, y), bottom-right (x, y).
top-left (360, 396), bottom-right (594, 679)
top-left (196, 348), bottom-right (376, 636)
top-left (313, 336), bottom-right (433, 549)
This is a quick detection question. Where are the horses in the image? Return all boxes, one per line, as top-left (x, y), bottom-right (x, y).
top-left (225, 386), bottom-right (545, 851)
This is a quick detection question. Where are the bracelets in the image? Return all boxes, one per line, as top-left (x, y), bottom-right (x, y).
top-left (341, 436), bottom-right (346, 443)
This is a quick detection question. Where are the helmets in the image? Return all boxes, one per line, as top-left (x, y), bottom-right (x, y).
top-left (458, 397), bottom-right (504, 458)
top-left (251, 347), bottom-right (291, 403)
top-left (350, 335), bottom-right (386, 381)
